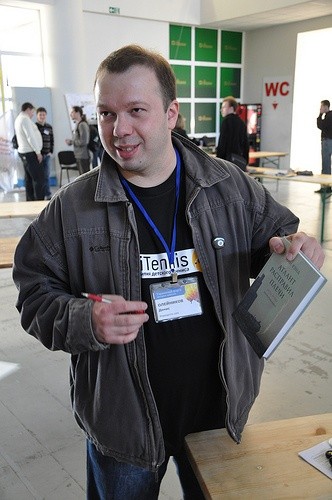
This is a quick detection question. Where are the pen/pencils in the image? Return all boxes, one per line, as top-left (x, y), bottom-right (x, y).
top-left (81, 292), bottom-right (146, 314)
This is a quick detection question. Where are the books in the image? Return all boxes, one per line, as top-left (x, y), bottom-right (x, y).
top-left (230, 238), bottom-right (327, 360)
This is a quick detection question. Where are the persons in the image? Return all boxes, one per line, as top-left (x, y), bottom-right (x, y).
top-left (65, 106), bottom-right (90, 175)
top-left (215, 96), bottom-right (249, 172)
top-left (14, 103), bottom-right (44, 201)
top-left (12, 44), bottom-right (324, 500)
top-left (175, 114), bottom-right (199, 146)
top-left (35, 107), bottom-right (55, 200)
top-left (315, 100), bottom-right (332, 194)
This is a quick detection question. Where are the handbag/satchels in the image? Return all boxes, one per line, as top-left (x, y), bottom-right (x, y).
top-left (231, 152), bottom-right (247, 171)
top-left (12, 135), bottom-right (18, 149)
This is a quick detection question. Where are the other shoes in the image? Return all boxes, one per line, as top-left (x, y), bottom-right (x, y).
top-left (44, 195), bottom-right (51, 200)
top-left (314, 187), bottom-right (331, 193)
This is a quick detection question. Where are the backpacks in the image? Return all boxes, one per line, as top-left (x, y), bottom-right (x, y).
top-left (76, 120), bottom-right (99, 153)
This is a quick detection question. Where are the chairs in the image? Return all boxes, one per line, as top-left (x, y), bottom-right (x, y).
top-left (57, 151), bottom-right (80, 188)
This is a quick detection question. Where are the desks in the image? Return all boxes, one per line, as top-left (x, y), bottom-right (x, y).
top-left (183, 414), bottom-right (332, 500)
top-left (0, 200), bottom-right (52, 268)
top-left (207, 152), bottom-right (332, 244)
